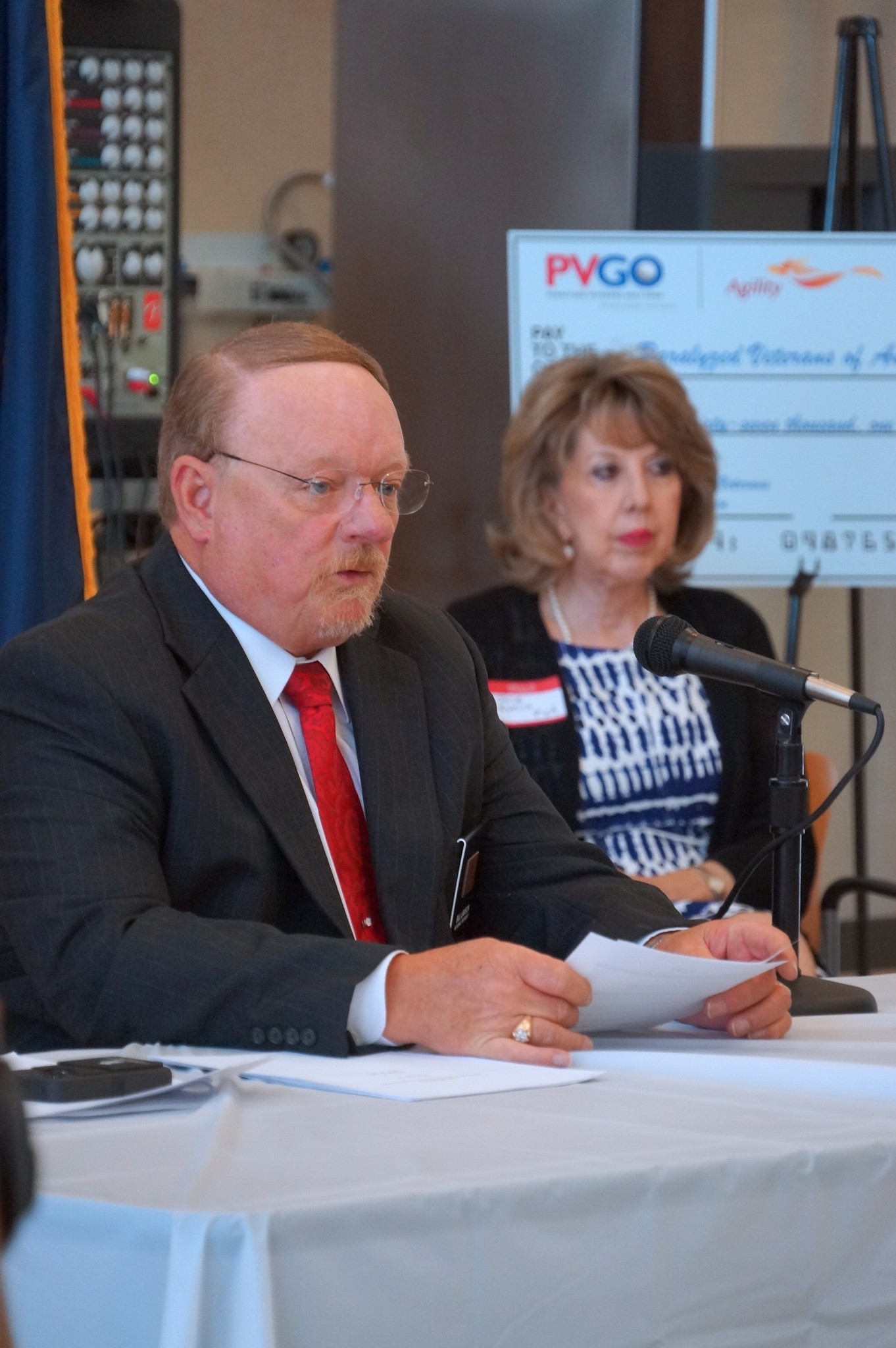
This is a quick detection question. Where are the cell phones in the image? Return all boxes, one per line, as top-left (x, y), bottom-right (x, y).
top-left (9, 1056), bottom-right (172, 1102)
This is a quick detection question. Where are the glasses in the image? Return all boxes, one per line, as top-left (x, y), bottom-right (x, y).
top-left (202, 448), bottom-right (435, 516)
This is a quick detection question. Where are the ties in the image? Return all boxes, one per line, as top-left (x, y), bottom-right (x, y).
top-left (281, 661), bottom-right (389, 942)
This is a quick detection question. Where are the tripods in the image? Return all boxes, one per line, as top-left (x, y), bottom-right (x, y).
top-left (783, 14), bottom-right (896, 978)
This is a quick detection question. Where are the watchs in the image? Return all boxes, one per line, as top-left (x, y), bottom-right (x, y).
top-left (696, 865), bottom-right (726, 905)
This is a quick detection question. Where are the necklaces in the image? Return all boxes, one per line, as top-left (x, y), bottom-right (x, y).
top-left (548, 584), bottom-right (658, 649)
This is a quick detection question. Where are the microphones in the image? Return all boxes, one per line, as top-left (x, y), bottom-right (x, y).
top-left (633, 613), bottom-right (881, 716)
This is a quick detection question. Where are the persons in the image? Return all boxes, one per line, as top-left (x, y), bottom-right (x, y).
top-left (0, 325), bottom-right (797, 1069)
top-left (445, 354), bottom-right (823, 973)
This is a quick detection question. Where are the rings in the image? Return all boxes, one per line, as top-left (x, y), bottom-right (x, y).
top-left (512, 1014), bottom-right (533, 1043)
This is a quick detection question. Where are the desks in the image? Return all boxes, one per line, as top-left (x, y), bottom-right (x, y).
top-left (0, 964), bottom-right (894, 1347)
